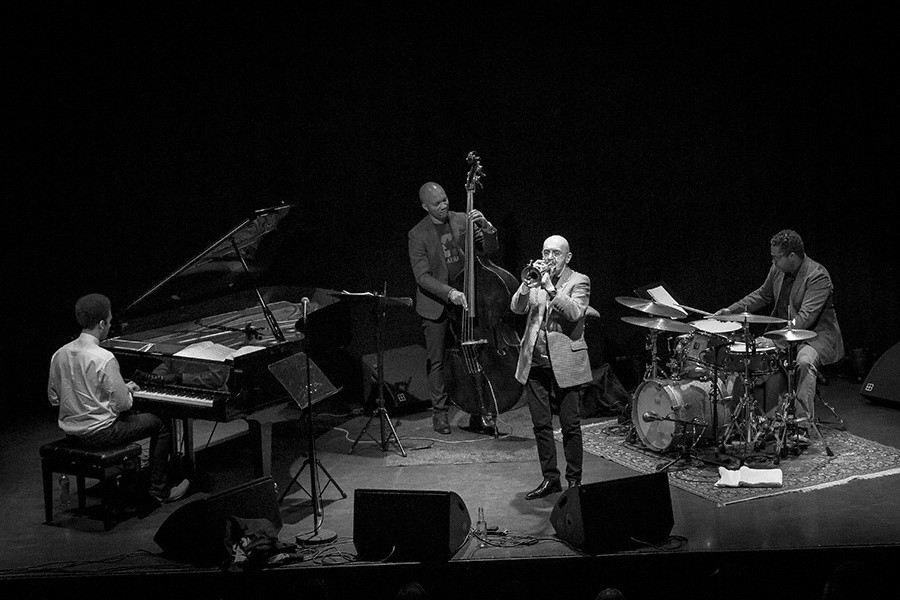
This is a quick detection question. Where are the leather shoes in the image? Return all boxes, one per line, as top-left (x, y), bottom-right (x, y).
top-left (569, 480), bottom-right (582, 488)
top-left (525, 479), bottom-right (562, 500)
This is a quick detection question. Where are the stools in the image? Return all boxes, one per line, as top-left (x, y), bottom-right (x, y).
top-left (40, 438), bottom-right (143, 531)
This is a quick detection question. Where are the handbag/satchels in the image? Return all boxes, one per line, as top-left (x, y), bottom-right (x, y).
top-left (577, 363), bottom-right (629, 420)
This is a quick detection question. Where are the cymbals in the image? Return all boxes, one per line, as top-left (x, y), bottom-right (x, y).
top-left (703, 313), bottom-right (789, 323)
top-left (616, 297), bottom-right (686, 318)
top-left (622, 318), bottom-right (694, 333)
top-left (762, 330), bottom-right (818, 343)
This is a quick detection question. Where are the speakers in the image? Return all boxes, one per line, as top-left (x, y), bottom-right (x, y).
top-left (859, 342), bottom-right (900, 406)
top-left (550, 472), bottom-right (675, 552)
top-left (153, 475), bottom-right (283, 563)
top-left (354, 488), bottom-right (473, 560)
top-left (361, 344), bottom-right (431, 412)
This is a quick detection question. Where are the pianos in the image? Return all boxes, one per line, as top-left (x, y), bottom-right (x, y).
top-left (442, 150), bottom-right (527, 438)
top-left (99, 203), bottom-right (377, 481)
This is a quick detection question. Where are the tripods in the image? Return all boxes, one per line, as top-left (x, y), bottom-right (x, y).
top-left (327, 282), bottom-right (408, 456)
top-left (656, 317), bottom-right (834, 472)
top-left (267, 321), bottom-right (343, 516)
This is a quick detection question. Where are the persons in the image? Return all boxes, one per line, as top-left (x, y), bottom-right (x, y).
top-left (716, 230), bottom-right (845, 420)
top-left (510, 235), bottom-right (601, 501)
top-left (48, 294), bottom-right (191, 505)
top-left (409, 182), bottom-right (495, 434)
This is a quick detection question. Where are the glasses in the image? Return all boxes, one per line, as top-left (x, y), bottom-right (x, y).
top-left (771, 254), bottom-right (785, 261)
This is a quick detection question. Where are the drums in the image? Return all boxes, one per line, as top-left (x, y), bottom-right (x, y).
top-left (677, 331), bottom-right (730, 376)
top-left (687, 319), bottom-right (746, 342)
top-left (631, 380), bottom-right (729, 454)
top-left (723, 343), bottom-right (782, 376)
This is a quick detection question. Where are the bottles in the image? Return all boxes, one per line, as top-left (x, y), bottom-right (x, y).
top-left (58, 473), bottom-right (71, 507)
top-left (476, 507), bottom-right (487, 548)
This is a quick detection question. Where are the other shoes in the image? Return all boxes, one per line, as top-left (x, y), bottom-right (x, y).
top-left (151, 478), bottom-right (189, 502)
top-left (798, 428), bottom-right (810, 438)
top-left (432, 414), bottom-right (451, 433)
top-left (470, 416), bottom-right (509, 435)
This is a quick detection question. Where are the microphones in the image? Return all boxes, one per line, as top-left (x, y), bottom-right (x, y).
top-left (300, 296), bottom-right (310, 323)
top-left (460, 339), bottom-right (489, 346)
top-left (641, 412), bottom-right (664, 422)
top-left (751, 340), bottom-right (759, 359)
